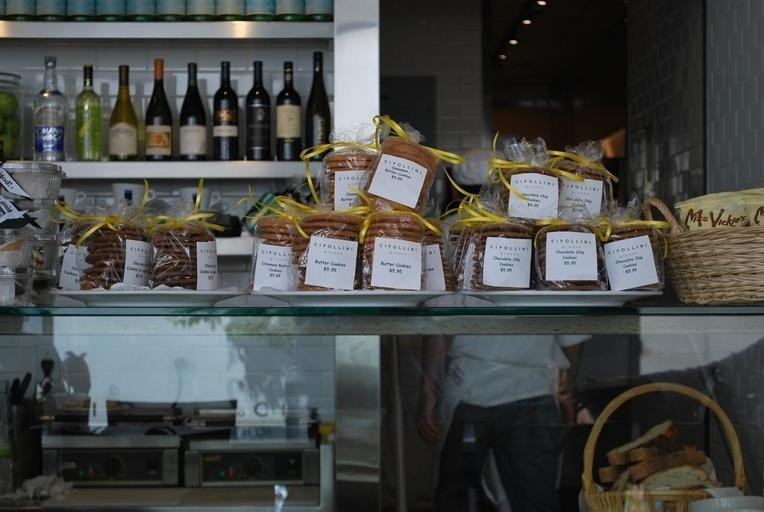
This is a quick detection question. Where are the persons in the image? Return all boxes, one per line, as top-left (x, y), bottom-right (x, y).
top-left (416, 148), bottom-right (575, 512)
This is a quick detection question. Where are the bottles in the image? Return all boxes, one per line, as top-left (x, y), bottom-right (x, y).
top-left (31, 52), bottom-right (330, 162)
top-left (0, 378), bottom-right (17, 496)
top-left (0, 71), bottom-right (23, 165)
top-left (1, 264), bottom-right (17, 307)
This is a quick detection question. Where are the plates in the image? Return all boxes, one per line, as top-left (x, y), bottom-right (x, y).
top-left (48, 286), bottom-right (248, 309)
top-left (248, 289), bottom-right (459, 309)
top-left (460, 287), bottom-right (666, 308)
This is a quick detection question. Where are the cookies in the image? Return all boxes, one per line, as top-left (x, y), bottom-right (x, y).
top-left (258, 136), bottom-right (663, 292)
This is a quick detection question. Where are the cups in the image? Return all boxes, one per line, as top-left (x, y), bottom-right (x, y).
top-left (60, 183), bottom-right (224, 214)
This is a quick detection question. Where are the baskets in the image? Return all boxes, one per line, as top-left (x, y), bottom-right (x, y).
top-left (581, 382), bottom-right (746, 512)
top-left (642, 196), bottom-right (763, 302)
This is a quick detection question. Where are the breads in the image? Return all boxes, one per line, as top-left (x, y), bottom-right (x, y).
top-left (72, 221), bottom-right (214, 290)
top-left (598, 419), bottom-right (722, 491)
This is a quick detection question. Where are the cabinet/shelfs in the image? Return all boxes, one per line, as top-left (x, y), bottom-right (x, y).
top-left (0, 0), bottom-right (379, 260)
top-left (0, 289), bottom-right (764, 511)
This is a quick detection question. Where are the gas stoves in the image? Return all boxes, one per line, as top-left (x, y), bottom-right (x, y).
top-left (37, 417), bottom-right (319, 487)
